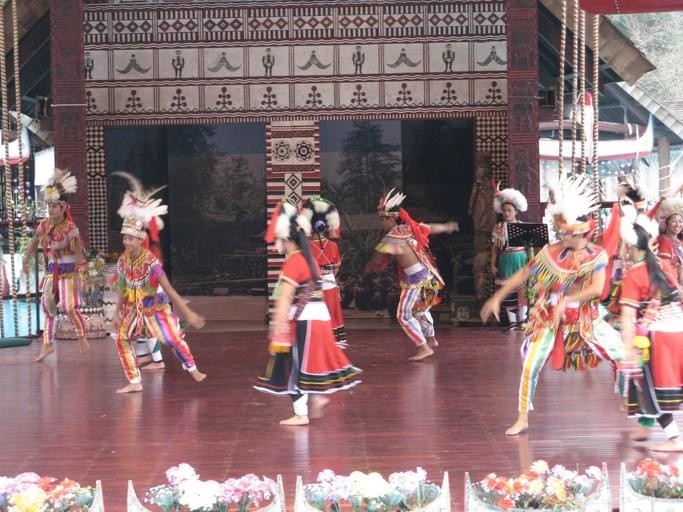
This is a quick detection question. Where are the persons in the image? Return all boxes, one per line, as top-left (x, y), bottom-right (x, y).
top-left (253, 205), bottom-right (361, 425)
top-left (479, 192), bottom-right (681, 452)
top-left (21, 197), bottom-right (91, 363)
top-left (105, 233), bottom-right (209, 395)
top-left (371, 206), bottom-right (459, 359)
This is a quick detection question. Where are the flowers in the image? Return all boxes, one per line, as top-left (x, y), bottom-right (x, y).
top-left (301, 463), bottom-right (439, 510)
top-left (143, 461), bottom-right (276, 512)
top-left (631, 457), bottom-right (683, 504)
top-left (0, 470), bottom-right (109, 510)
top-left (474, 459), bottom-right (605, 511)
